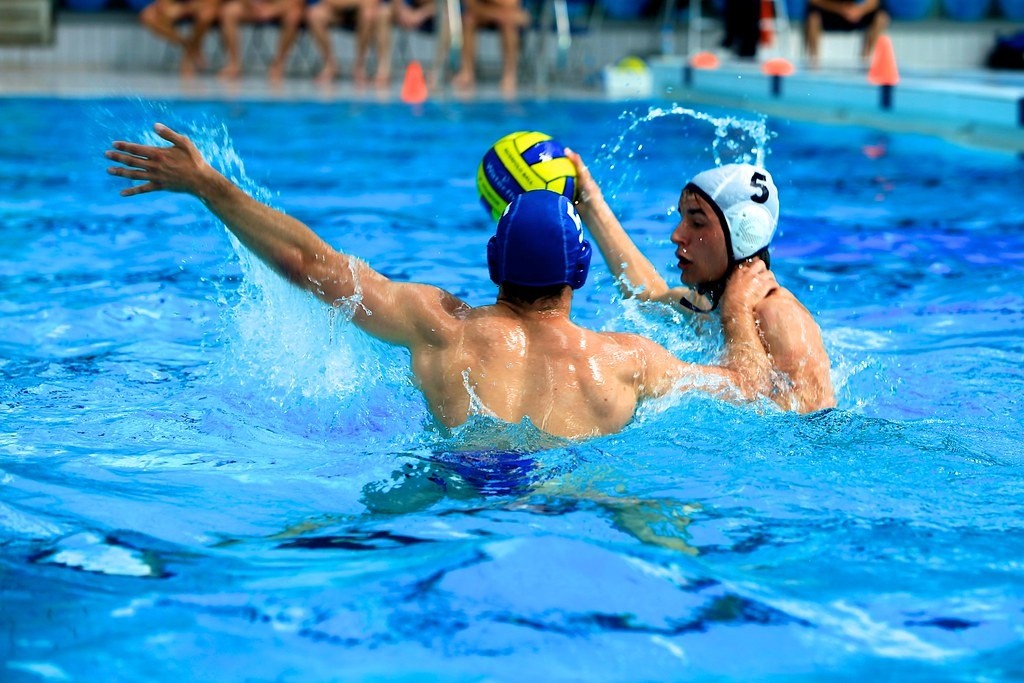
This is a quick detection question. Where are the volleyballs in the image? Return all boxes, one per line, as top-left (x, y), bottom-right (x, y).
top-left (476, 130), bottom-right (577, 224)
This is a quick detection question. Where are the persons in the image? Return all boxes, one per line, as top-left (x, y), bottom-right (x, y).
top-left (105, 122), bottom-right (775, 446)
top-left (217, 0), bottom-right (301, 87)
top-left (141, 0), bottom-right (217, 86)
top-left (451, 1), bottom-right (530, 95)
top-left (806, 0), bottom-right (890, 71)
top-left (559, 141), bottom-right (835, 414)
top-left (307, 1), bottom-right (371, 84)
top-left (373, 0), bottom-right (450, 90)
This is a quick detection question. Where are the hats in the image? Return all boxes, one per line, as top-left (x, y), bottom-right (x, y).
top-left (487, 190), bottom-right (592, 289)
top-left (686, 163), bottom-right (778, 264)
